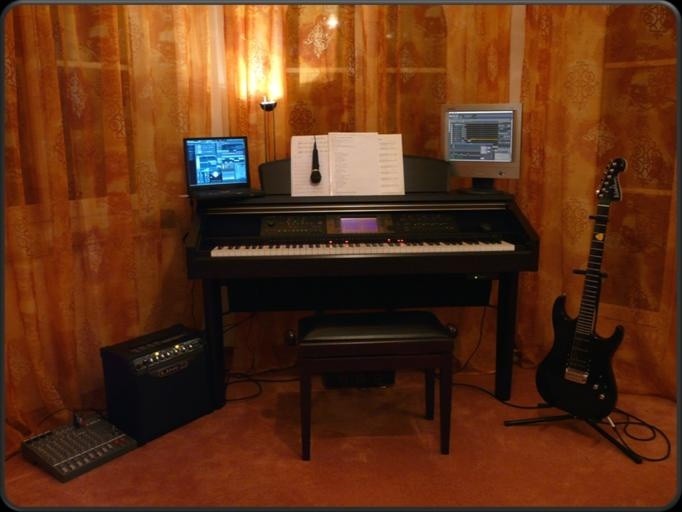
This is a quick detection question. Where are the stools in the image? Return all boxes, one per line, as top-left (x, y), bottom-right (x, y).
top-left (295, 309), bottom-right (454, 460)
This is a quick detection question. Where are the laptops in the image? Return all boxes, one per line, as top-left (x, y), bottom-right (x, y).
top-left (184, 136), bottom-right (266, 201)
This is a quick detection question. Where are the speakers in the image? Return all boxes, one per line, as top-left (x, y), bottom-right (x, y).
top-left (97, 323), bottom-right (223, 445)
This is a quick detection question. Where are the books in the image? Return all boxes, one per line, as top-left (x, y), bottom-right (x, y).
top-left (376, 133), bottom-right (406, 197)
top-left (290, 133), bottom-right (330, 198)
top-left (329, 130), bottom-right (381, 197)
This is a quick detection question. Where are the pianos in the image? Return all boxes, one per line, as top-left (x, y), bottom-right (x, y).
top-left (195, 155), bottom-right (539, 401)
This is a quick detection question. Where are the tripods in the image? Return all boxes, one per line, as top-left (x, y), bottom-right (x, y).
top-left (504, 268), bottom-right (641, 464)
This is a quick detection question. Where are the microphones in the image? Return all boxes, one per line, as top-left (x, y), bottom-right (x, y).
top-left (311, 142), bottom-right (320, 184)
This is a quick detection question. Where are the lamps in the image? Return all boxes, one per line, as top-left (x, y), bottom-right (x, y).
top-left (258, 100), bottom-right (279, 164)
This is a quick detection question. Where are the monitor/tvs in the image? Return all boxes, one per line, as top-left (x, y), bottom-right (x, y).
top-left (439, 103), bottom-right (523, 195)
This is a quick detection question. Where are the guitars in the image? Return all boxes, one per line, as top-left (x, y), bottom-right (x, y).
top-left (535, 157), bottom-right (627, 419)
